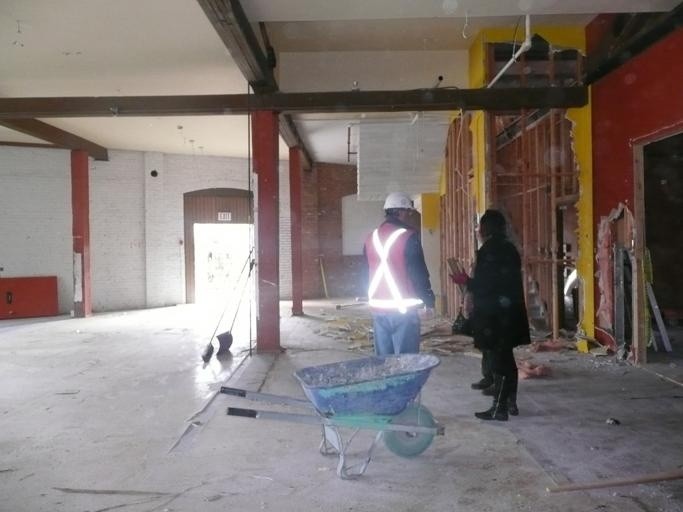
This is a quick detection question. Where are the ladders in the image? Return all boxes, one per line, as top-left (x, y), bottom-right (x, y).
top-left (624, 249), bottom-right (673, 352)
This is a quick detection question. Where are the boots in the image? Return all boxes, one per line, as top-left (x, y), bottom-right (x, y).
top-left (475, 375), bottom-right (508, 420)
top-left (507, 368), bottom-right (519, 416)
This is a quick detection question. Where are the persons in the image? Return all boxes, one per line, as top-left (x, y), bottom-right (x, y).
top-left (448, 223), bottom-right (496, 398)
top-left (468, 209), bottom-right (531, 421)
top-left (361, 191), bottom-right (436, 355)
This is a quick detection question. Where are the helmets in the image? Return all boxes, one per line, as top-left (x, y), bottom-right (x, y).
top-left (383, 192), bottom-right (416, 211)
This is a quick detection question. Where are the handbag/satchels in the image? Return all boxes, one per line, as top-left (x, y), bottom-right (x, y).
top-left (451, 313), bottom-right (475, 336)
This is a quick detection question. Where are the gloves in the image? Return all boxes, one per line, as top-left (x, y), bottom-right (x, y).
top-left (448, 268), bottom-right (469, 284)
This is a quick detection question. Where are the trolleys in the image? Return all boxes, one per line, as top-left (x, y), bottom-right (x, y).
top-left (221, 352), bottom-right (444, 482)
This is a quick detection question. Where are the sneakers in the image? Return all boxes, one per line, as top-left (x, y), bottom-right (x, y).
top-left (471, 376), bottom-right (496, 395)
